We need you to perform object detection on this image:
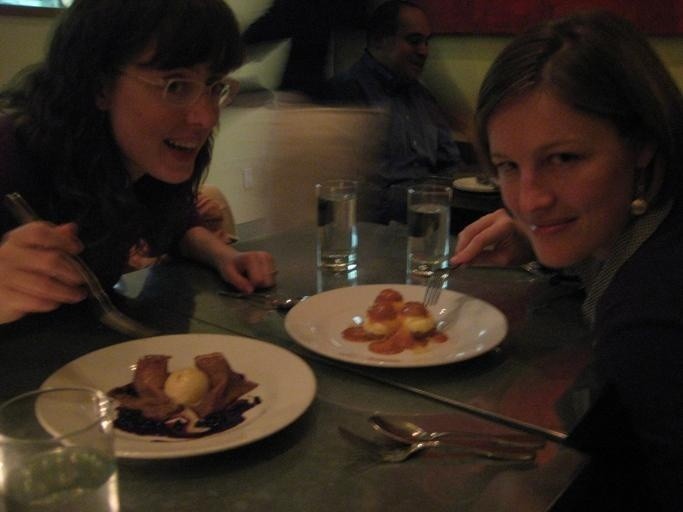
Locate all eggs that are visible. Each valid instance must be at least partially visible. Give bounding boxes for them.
[161,366,212,406]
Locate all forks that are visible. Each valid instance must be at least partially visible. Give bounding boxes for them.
[1,192,158,338]
[423,258,467,305]
[336,424,534,465]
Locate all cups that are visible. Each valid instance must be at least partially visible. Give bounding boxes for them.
[0,386,118,511]
[313,178,360,273]
[404,183,454,279]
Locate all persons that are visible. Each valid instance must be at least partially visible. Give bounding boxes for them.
[319,1,477,231]
[445,9,681,508]
[0,1,277,347]
[240,0,374,93]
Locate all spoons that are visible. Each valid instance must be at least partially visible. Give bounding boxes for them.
[367,414,548,451]
[217,287,299,309]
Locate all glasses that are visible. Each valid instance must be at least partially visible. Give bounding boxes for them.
[122,70,240,109]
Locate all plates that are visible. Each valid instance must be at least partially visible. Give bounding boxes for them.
[35,333,318,459]
[287,282,508,368]
[453,176,498,192]
